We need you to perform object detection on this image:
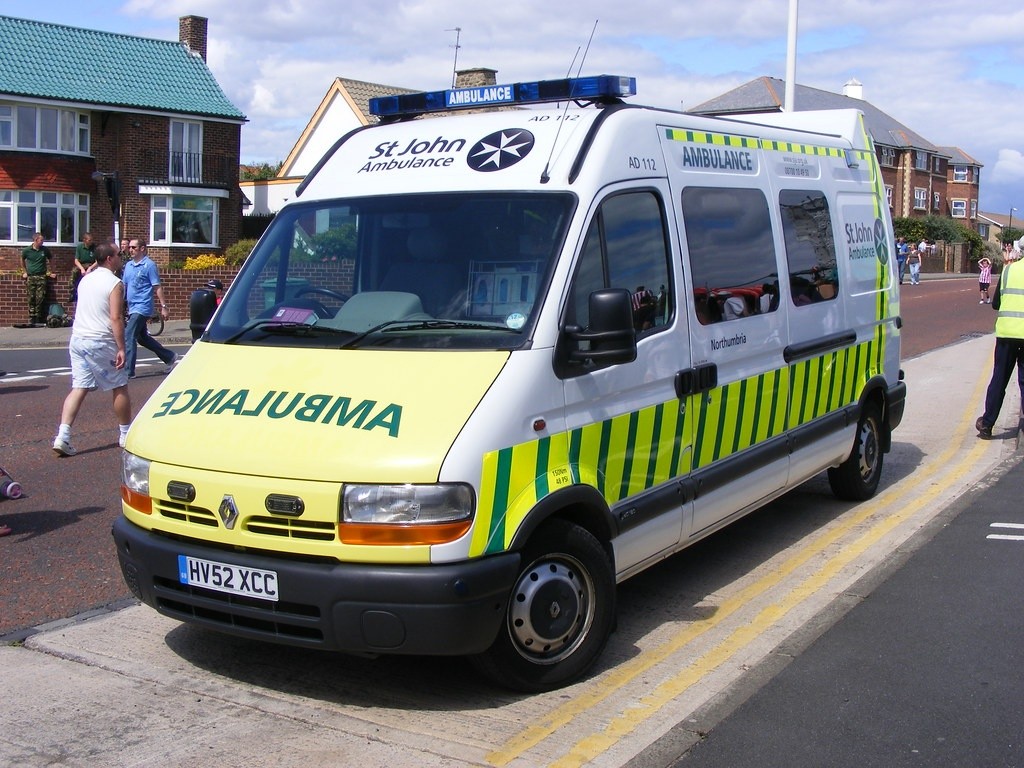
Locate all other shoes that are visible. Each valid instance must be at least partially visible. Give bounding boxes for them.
[916,281,919,285]
[979,300,984,304]
[976,416,993,440]
[910,282,913,285]
[0,524,13,536]
[986,297,990,304]
[161,351,179,373]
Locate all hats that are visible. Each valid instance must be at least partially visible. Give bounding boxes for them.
[523,206,556,226]
[1013,235,1024,257]
[203,279,223,290]
[716,291,728,295]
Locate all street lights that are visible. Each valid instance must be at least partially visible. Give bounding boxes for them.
[1009,207,1018,244]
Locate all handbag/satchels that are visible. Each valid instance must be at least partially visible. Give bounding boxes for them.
[992,275,1002,310]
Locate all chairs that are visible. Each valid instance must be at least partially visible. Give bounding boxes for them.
[377,225,463,311]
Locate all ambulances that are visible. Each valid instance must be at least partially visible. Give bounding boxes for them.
[107,73,908,695]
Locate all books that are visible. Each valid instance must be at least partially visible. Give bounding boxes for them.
[267,307,319,328]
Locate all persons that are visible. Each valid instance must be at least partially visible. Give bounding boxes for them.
[53,241,132,456]
[790,266,837,306]
[631,284,666,333]
[21,232,56,327]
[67,232,133,321]
[976,237,1024,439]
[120,238,178,379]
[203,279,224,307]
[978,242,1023,304]
[918,239,936,258]
[692,280,779,320]
[894,236,921,285]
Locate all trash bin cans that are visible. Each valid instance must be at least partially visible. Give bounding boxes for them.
[262,278,307,310]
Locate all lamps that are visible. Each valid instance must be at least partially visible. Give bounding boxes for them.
[92,169,117,182]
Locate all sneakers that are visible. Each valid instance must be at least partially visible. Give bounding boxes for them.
[118,435,127,448]
[52,437,78,455]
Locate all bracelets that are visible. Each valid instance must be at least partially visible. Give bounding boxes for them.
[161,305,167,308]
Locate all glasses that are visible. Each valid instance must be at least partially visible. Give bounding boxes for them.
[128,245,137,249]
